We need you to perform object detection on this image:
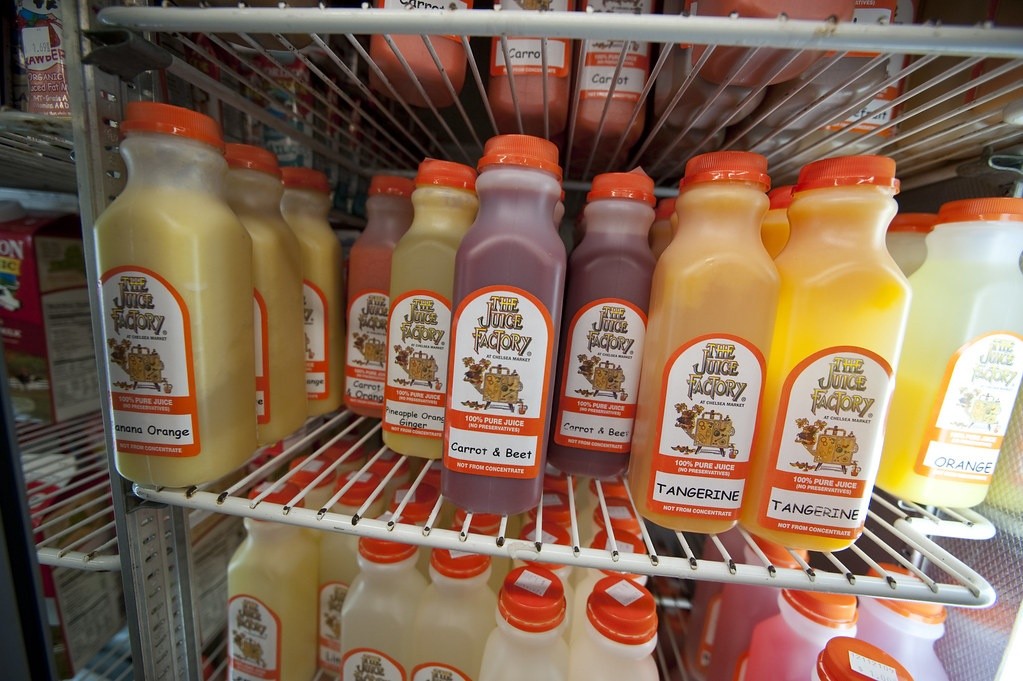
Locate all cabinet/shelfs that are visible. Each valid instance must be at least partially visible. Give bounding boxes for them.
[0,0,1023,681]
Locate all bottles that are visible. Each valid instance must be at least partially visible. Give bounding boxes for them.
[92,0,1023,681]
